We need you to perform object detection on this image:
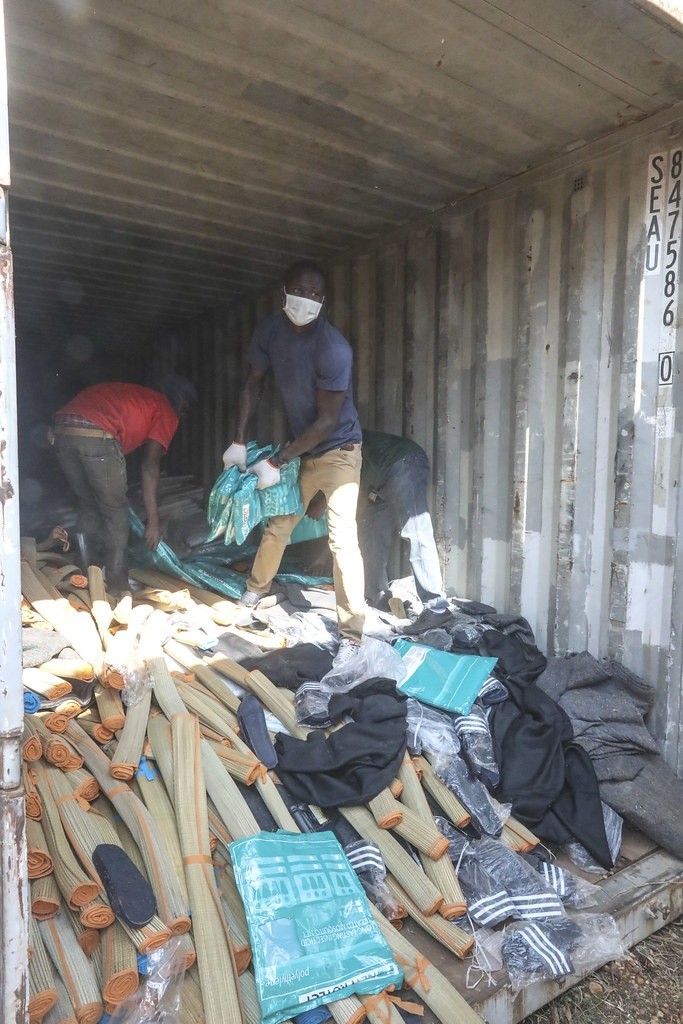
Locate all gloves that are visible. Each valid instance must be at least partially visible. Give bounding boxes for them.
[246,458,282,490]
[222,441,247,473]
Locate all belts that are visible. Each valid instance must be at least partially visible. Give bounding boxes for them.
[53,426,113,438]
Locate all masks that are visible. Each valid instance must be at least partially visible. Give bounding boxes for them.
[281,284,326,327]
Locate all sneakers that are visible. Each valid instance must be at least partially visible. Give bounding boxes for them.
[331,638,359,669]
[241,590,260,605]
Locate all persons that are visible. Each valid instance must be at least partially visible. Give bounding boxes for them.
[305,431,453,637]
[49,373,198,592]
[221,267,362,666]
